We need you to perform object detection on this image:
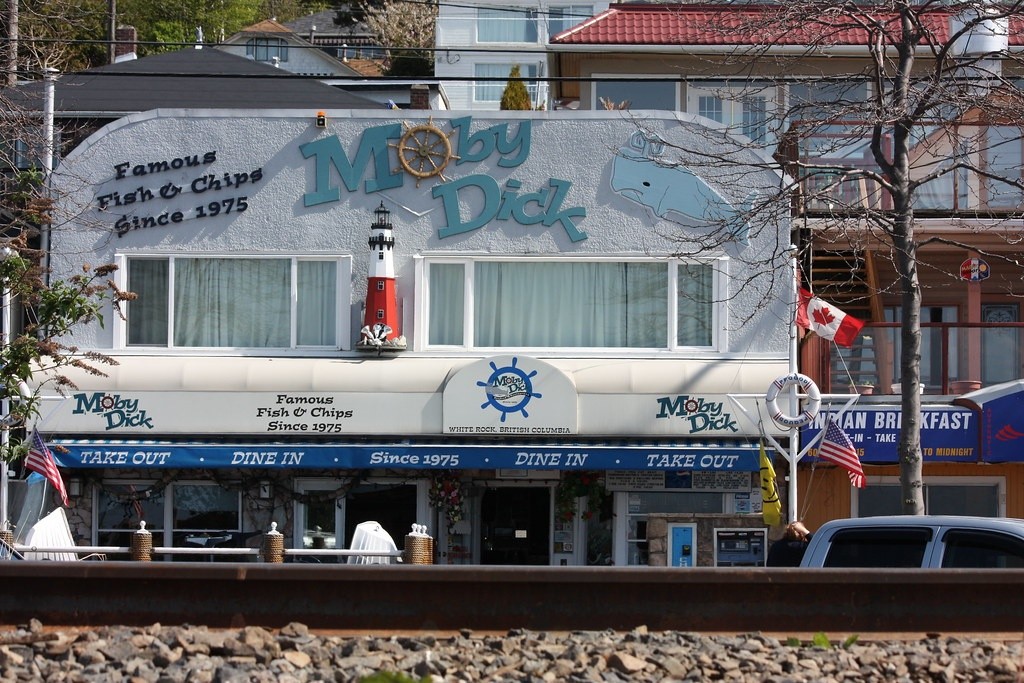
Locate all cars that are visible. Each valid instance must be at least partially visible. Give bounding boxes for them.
[799,514,1024,568]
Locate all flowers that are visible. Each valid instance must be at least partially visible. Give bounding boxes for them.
[554,469,605,523]
[423,468,466,531]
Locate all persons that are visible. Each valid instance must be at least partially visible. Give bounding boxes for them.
[766,522,813,567]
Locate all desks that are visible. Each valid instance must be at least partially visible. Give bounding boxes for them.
[185,534,233,562]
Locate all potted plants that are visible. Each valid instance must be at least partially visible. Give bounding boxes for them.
[848,380,875,395]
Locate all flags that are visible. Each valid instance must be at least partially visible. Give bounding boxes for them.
[760,438,782,527]
[817,418,867,490]
[23,425,70,508]
[128,480,144,519]
[794,287,865,349]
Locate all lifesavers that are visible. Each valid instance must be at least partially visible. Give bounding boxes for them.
[765,373,823,427]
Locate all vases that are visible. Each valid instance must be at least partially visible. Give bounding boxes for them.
[950,380,982,394]
[566,484,589,498]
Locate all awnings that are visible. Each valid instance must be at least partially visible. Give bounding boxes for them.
[45,439,775,474]
